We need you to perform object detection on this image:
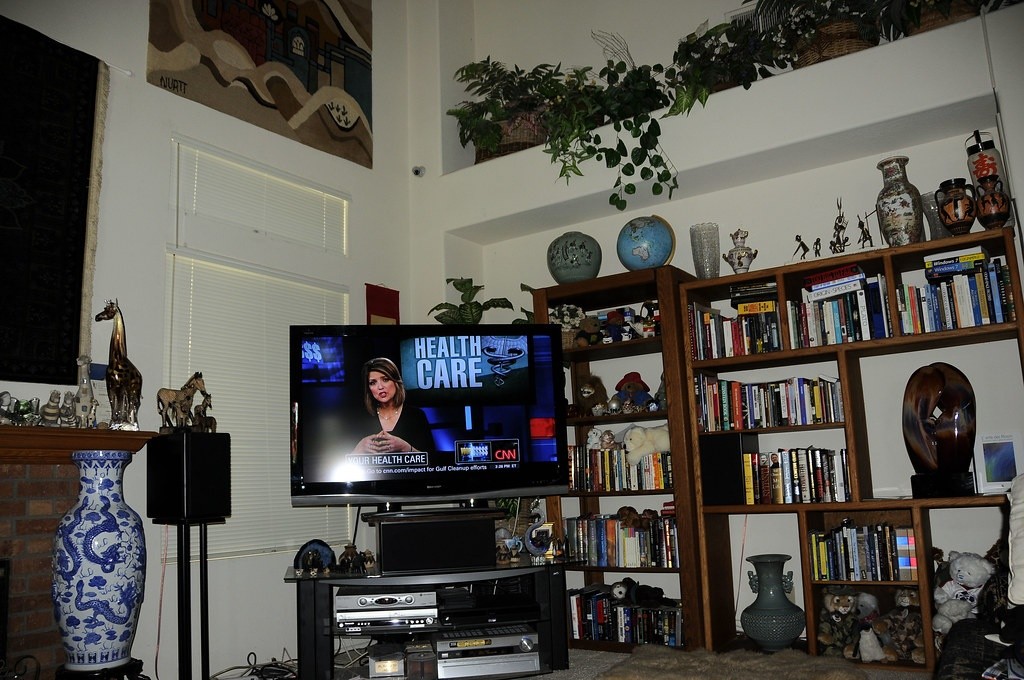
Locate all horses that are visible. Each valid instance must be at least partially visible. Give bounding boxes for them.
[156,372,218,433]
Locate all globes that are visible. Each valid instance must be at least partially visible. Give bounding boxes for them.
[617,215,676,271]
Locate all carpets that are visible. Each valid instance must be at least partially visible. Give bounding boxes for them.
[334,643,933,680]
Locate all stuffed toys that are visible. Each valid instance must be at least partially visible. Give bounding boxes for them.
[617,506,639,529]
[577,376,609,417]
[604,311,632,344]
[587,428,618,449]
[931,549,995,635]
[609,372,655,414]
[572,319,604,347]
[609,577,677,609]
[624,424,671,465]
[640,510,658,530]
[812,585,925,665]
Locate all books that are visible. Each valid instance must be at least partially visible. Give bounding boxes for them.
[687,244,1017,362]
[694,372,844,432]
[567,583,684,647]
[742,444,852,506]
[567,445,673,492]
[807,517,919,583]
[561,499,679,568]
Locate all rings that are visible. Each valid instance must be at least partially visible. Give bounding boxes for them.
[387,445,391,449]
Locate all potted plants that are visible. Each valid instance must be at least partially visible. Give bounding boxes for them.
[446,0,1001,211]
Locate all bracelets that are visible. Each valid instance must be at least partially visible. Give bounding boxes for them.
[411,445,413,452]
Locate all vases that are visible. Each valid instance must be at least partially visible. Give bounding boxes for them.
[874,156,922,248]
[689,222,721,279]
[52,450,147,671]
[740,554,805,651]
[920,190,953,241]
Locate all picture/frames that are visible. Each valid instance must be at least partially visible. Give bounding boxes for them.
[978,432,1021,493]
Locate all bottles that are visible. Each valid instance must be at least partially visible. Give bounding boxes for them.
[875,129,1012,248]
[52,450,147,671]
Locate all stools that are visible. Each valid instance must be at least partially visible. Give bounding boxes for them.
[933,619,1006,680]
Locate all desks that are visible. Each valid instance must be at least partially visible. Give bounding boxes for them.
[0,423,164,680]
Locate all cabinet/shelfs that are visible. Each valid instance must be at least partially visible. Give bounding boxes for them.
[679,226,1024,671]
[531,265,705,654]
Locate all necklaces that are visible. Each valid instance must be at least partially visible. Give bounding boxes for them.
[376,404,398,420]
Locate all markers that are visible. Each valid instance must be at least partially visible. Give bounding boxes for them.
[449,639,492,647]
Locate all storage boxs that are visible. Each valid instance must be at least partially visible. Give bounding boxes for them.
[367,645,437,677]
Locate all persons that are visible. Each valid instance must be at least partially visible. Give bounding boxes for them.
[771,453,779,468]
[343,358,439,469]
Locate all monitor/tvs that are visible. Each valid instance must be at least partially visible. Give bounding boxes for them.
[290,324,569,521]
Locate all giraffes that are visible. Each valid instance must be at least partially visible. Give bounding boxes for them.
[95,298,143,430]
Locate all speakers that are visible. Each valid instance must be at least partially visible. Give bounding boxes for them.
[699,432,759,507]
[146,431,232,521]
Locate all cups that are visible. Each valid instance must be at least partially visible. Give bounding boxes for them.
[918,188,955,240]
[690,223,721,281]
